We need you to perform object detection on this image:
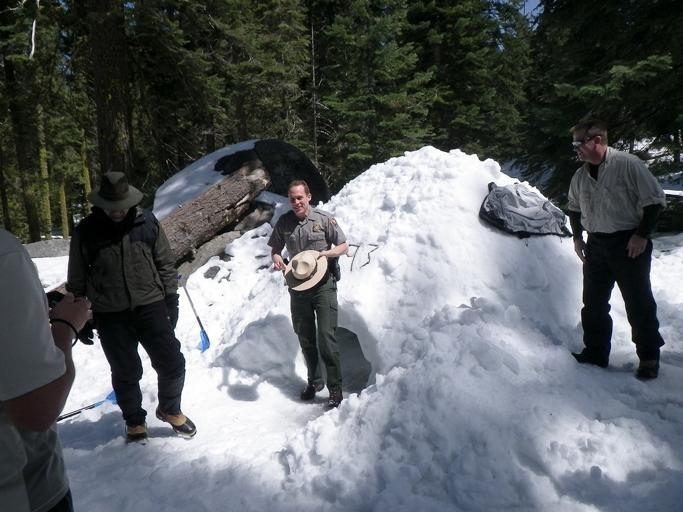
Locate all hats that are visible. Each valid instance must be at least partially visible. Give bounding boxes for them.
[285,250,328,292]
[87,172,143,209]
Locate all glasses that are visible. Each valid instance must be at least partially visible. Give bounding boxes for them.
[573,137,594,148]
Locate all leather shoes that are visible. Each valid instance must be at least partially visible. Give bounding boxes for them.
[328,390,343,406]
[303,381,323,399]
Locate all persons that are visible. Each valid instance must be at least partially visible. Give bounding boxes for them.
[265,180,349,408]
[64,172,196,445]
[566,119,667,382]
[0,227,92,511]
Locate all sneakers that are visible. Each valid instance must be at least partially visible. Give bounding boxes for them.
[637,360,659,379]
[126,425,147,441]
[155,407,197,435]
[572,352,609,367]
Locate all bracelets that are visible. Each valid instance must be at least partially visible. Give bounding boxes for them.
[48,316,79,346]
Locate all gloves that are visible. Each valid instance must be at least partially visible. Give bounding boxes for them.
[164,294,179,328]
[78,322,95,346]
